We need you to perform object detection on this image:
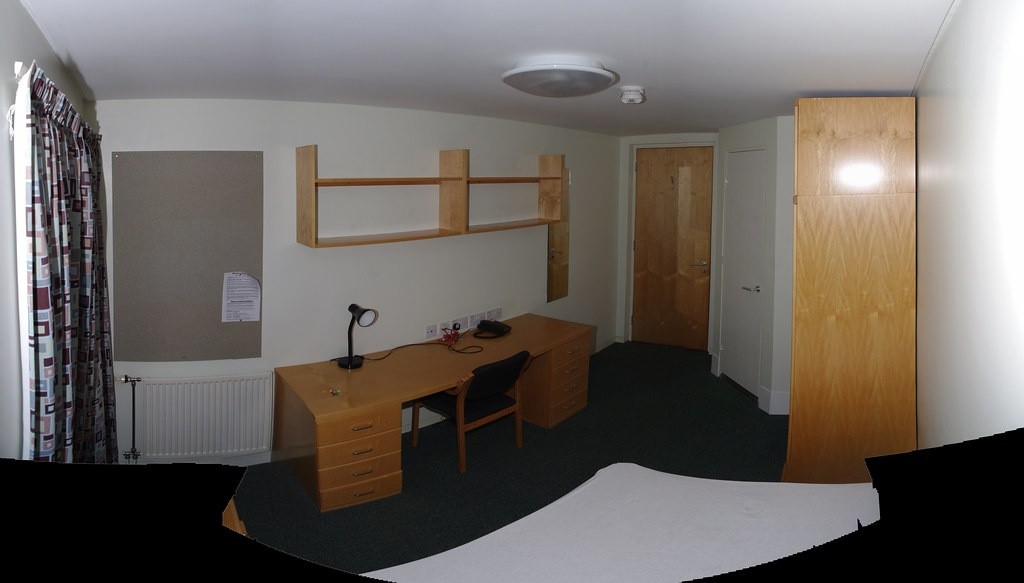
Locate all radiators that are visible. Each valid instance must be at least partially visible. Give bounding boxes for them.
[140,370,275,457]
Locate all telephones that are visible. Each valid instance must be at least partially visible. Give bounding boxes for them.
[477,319,512,335]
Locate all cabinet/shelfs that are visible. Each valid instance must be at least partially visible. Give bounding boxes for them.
[785,96,917,484]
[295,144,565,248]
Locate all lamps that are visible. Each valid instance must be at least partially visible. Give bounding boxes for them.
[501,55,615,98]
[336,303,375,369]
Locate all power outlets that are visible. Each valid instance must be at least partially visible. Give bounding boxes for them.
[451,316,469,330]
[425,323,438,338]
[486,308,502,321]
[469,312,486,327]
[438,321,451,335]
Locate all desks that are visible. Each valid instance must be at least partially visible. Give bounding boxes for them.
[275,313,595,513]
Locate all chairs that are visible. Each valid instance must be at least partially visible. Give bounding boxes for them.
[411,350,534,474]
